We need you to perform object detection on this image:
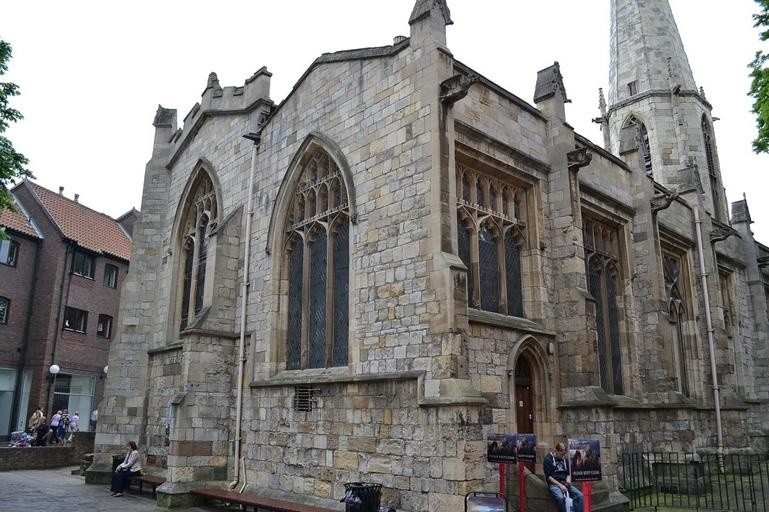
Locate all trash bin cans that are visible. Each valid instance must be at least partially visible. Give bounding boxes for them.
[110,454,132,492]
[339,481,384,512]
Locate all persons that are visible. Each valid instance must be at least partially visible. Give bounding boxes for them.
[27,405,81,446]
[110,440,141,497]
[543,442,584,511]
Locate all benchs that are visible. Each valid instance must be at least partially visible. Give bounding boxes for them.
[129,475,166,499]
[189,489,342,512]
